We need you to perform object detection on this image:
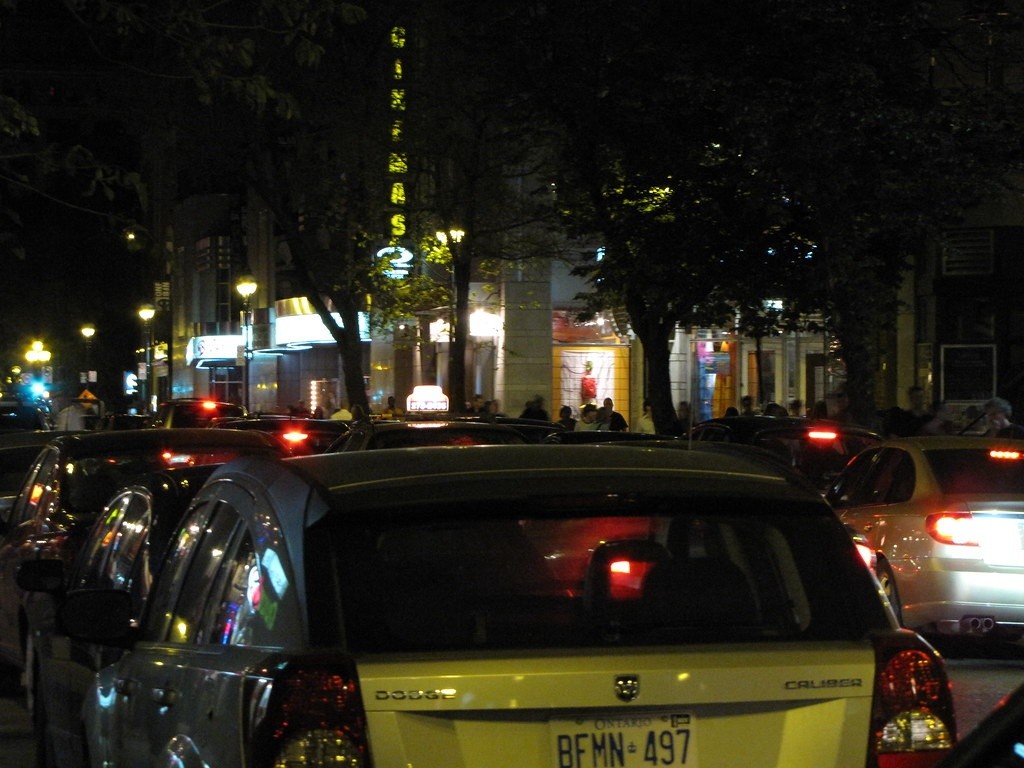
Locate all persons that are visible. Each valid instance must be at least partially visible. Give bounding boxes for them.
[983,397,1024,439]
[518,394,551,422]
[555,404,579,430]
[282,390,337,419]
[882,385,943,438]
[723,406,739,416]
[383,396,406,421]
[603,397,629,432]
[810,399,827,419]
[791,399,802,416]
[579,359,597,402]
[574,403,598,431]
[634,398,655,434]
[587,407,609,430]
[451,395,508,417]
[962,406,987,432]
[762,403,789,417]
[677,400,698,431]
[742,395,758,416]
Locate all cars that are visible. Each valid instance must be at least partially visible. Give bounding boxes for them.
[55,437,962,768]
[0,384,664,768]
[674,411,888,498]
[821,434,1024,647]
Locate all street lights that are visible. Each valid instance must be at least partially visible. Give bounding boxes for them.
[436,226,468,413]
[136,303,160,413]
[79,322,97,390]
[234,276,260,415]
